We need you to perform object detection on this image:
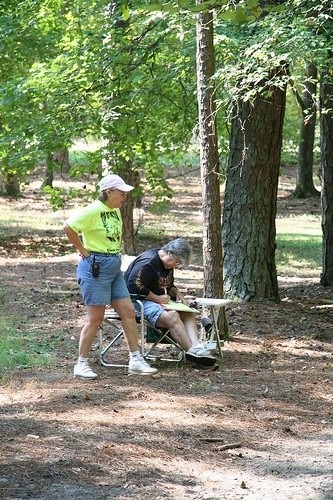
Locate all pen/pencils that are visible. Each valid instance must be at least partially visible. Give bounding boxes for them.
[165,288,167,294]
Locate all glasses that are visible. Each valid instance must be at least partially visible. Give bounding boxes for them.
[175,258,185,269]
[109,192,129,196]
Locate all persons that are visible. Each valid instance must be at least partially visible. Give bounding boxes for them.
[121,238,220,371]
[63,174,158,380]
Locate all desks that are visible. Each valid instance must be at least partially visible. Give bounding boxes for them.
[177,298,230,357]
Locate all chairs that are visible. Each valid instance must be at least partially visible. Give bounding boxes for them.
[98,271,185,368]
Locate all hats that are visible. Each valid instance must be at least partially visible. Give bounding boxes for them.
[100,175,134,192]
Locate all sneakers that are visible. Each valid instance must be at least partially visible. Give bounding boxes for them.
[127,357,158,375]
[73,363,97,379]
[184,346,219,371]
